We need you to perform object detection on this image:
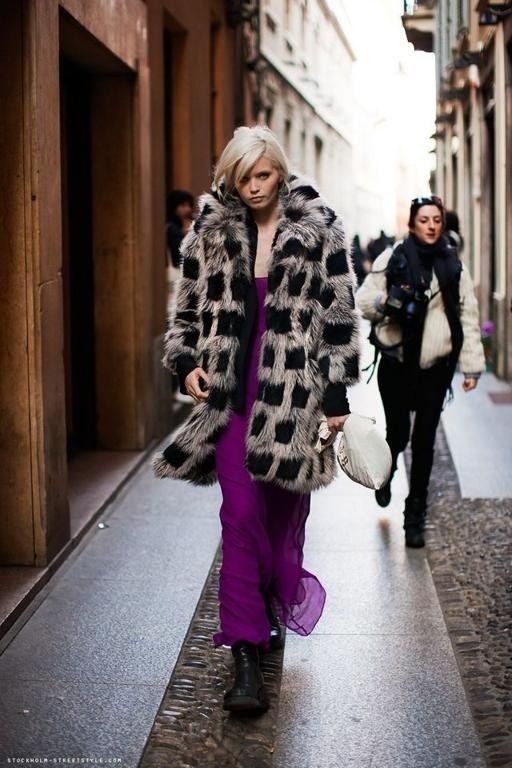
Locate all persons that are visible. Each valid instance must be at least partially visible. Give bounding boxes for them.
[444,210,462,249]
[160,123,362,715]
[349,229,397,289]
[164,185,199,320]
[354,195,489,547]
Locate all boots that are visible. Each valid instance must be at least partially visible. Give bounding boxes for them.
[375,465,398,509]
[222,618,285,713]
[404,498,428,548]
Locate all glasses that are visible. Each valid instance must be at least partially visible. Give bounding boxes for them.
[412,196,443,205]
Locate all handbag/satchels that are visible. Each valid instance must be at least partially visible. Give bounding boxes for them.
[313,414,393,490]
[367,240,407,363]
